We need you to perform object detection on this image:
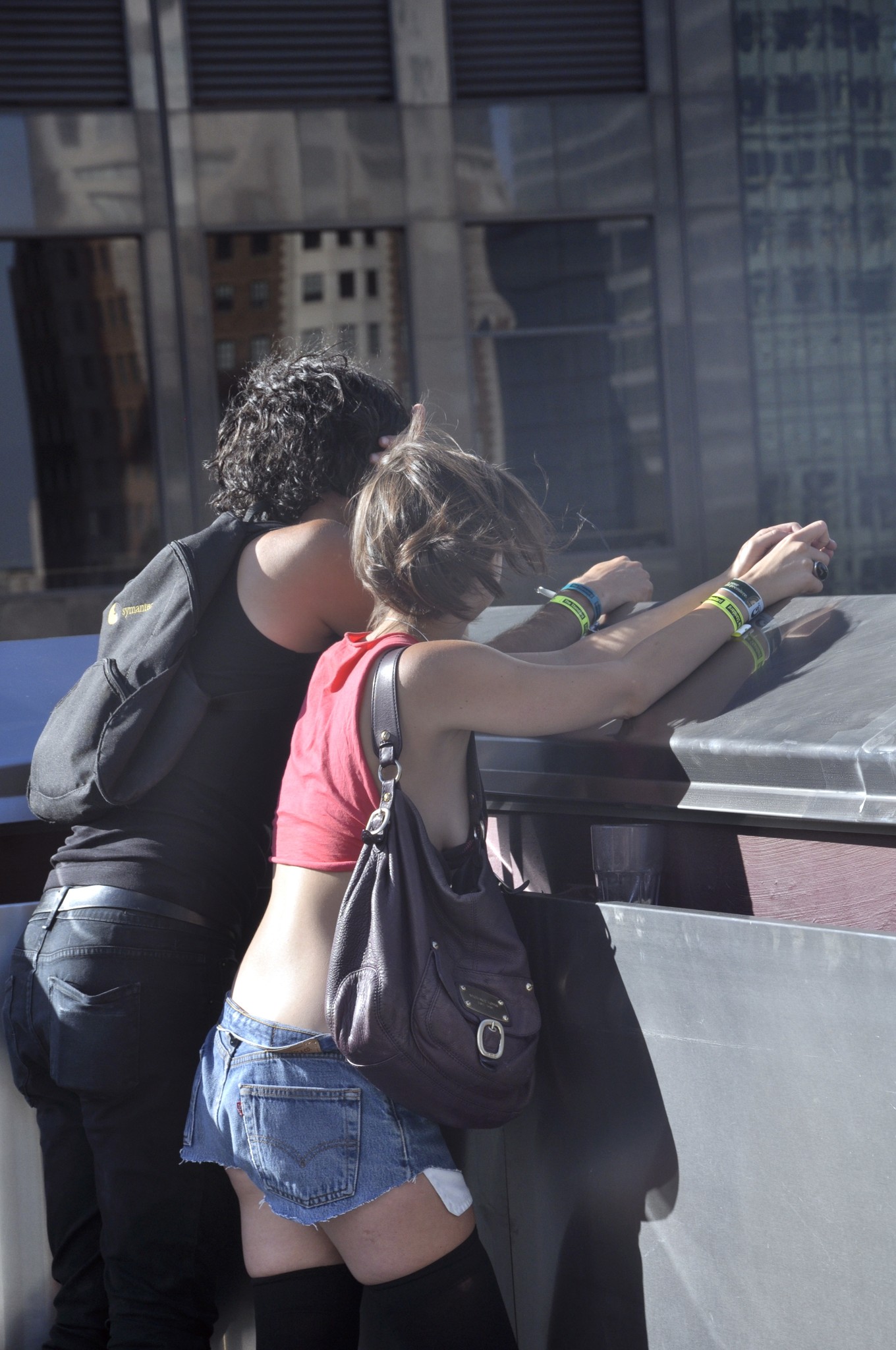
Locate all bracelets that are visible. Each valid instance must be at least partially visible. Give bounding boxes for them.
[702,593,752,637]
[748,612,782,660]
[560,583,601,621]
[548,595,590,638]
[719,577,764,622]
[734,629,765,674]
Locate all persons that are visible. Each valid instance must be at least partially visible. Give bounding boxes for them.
[179,406,838,1350]
[0,341,654,1350]
[623,598,835,743]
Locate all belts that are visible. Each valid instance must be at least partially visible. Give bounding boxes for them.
[28,883,212,925]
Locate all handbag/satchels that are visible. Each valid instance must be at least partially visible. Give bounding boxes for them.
[327,645,540,1130]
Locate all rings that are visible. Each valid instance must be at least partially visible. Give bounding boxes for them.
[812,560,829,584]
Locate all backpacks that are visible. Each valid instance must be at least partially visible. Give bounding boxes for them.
[27,509,274,823]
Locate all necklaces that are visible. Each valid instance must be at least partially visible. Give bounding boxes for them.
[386,613,426,643]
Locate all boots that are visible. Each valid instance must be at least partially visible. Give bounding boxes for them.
[251,1263,358,1350]
[357,1223,519,1350]
